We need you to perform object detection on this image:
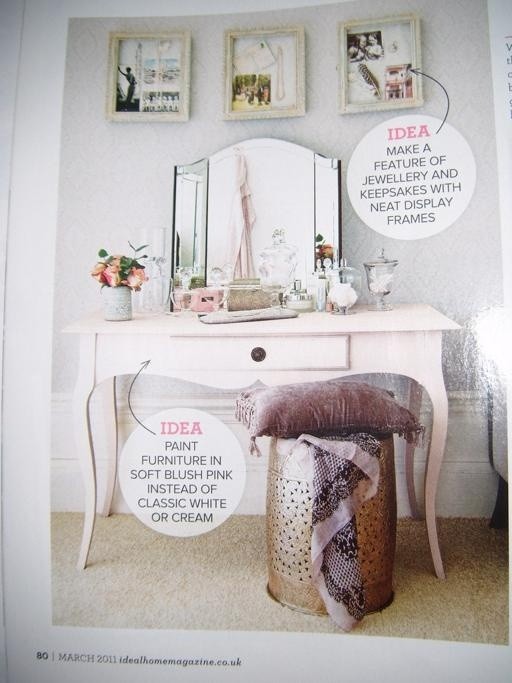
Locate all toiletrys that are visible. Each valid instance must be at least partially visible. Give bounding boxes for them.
[284,256,340,313]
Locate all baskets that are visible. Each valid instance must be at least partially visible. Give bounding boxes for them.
[188,285,277,312]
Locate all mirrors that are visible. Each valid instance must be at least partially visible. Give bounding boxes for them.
[168,137,341,305]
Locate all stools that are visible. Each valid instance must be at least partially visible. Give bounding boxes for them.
[263,435,398,618]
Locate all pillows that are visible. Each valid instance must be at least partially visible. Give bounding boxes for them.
[237,382,425,453]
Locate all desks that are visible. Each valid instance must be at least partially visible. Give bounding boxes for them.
[76,304,462,579]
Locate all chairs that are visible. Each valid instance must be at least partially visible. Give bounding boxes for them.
[469,304,509,530]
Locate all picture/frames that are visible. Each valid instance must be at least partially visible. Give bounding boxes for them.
[338,15,423,114]
[224,29,306,121]
[108,31,192,122]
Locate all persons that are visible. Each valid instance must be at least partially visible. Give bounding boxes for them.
[347,34,368,63]
[363,32,383,61]
[115,63,136,113]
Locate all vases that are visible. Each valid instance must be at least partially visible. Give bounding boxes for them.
[102,286,133,321]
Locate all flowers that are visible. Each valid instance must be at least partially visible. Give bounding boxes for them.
[90,240,149,292]
